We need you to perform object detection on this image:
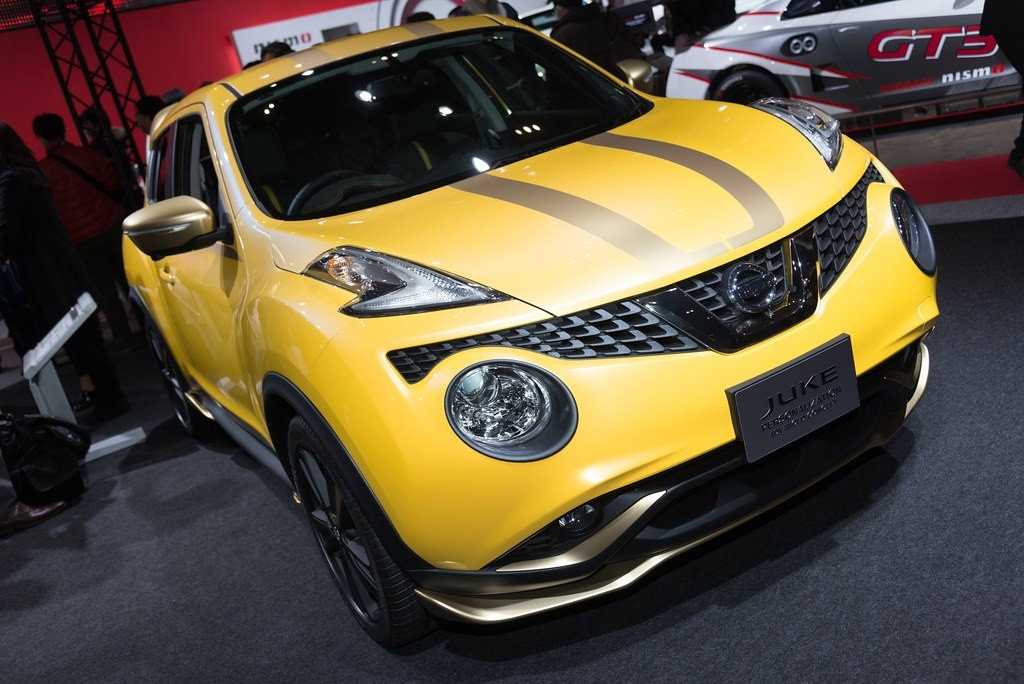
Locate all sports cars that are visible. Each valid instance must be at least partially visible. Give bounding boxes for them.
[666,1,1021,143]
[120,12,944,659]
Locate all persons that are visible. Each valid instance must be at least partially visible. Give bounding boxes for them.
[1,42,293,427]
[979,0,1024,174]
[408,0,737,84]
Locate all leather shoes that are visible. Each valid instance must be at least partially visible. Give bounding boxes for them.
[0,501,67,538]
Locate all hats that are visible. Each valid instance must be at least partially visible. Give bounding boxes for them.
[547,0,583,7]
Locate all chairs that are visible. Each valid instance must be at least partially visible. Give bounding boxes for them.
[255,108,383,215]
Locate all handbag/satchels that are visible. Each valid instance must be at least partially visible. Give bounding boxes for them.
[0,413,92,505]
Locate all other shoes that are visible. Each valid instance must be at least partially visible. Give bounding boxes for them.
[73,389,97,412]
